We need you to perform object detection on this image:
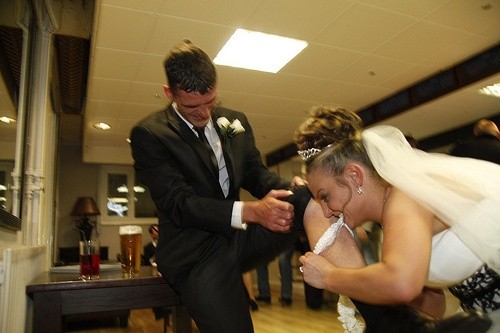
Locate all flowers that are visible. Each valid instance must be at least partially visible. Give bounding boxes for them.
[217,117,245,152]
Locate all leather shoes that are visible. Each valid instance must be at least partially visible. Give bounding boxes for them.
[364,313,493,333]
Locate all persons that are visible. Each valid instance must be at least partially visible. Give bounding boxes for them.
[129,40,500,333]
[293,105,500,333]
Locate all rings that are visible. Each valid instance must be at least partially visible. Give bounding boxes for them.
[299,265,305,274]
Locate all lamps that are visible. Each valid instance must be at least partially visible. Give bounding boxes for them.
[70,196,101,242]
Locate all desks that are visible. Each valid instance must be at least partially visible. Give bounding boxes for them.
[26,263,193,333]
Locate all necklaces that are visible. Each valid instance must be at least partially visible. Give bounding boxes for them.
[379,183,390,228]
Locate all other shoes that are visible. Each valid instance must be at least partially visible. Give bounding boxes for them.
[256,297,270,301]
[282,296,291,304]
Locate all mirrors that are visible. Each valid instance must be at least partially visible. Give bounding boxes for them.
[0,0,39,232]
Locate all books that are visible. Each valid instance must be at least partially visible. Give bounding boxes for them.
[51,265,122,273]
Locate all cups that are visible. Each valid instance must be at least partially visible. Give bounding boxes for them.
[79,240,100,279]
[119,225,143,278]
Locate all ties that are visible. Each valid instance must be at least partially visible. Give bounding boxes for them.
[192,126,219,185]
